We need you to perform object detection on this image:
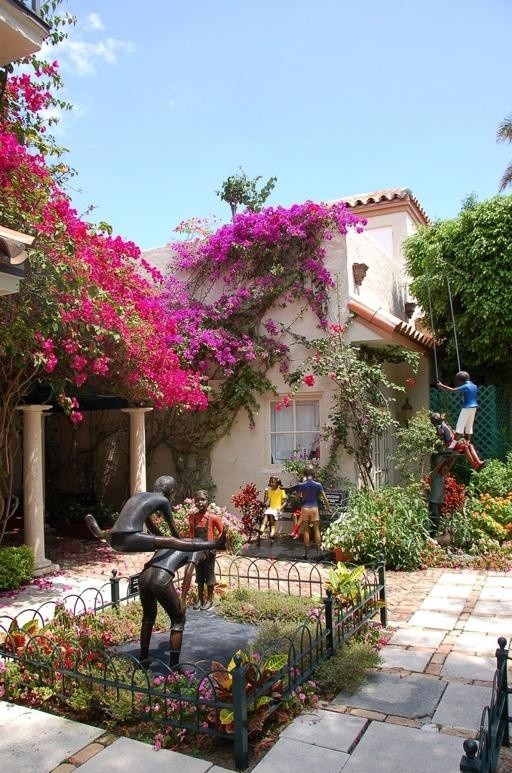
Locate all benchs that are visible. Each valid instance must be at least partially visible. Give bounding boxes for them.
[257,485,348,550]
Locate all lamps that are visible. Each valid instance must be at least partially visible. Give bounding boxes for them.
[401,396,413,429]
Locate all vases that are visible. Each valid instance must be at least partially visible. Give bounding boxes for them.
[334,547,353,566]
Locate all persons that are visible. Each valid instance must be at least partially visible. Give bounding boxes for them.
[139,537,213,674]
[84,475,227,553]
[188,490,223,611]
[431,412,487,472]
[288,476,307,540]
[279,463,332,562]
[436,370,478,442]
[427,456,457,538]
[258,477,288,539]
